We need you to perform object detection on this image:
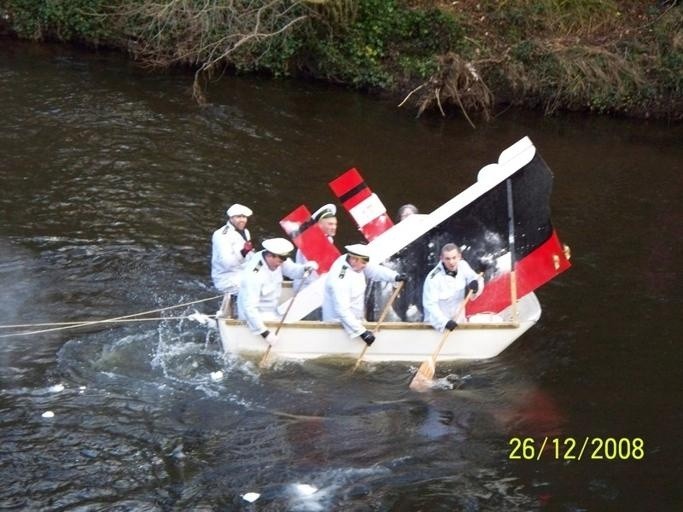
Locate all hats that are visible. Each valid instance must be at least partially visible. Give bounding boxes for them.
[262,238,294,256]
[344,244,373,258]
[311,203,336,222]
[227,203,253,217]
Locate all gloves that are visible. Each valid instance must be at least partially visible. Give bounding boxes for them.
[360,331,375,345]
[395,273,408,282]
[445,320,457,329]
[305,262,318,271]
[266,332,276,345]
[470,281,478,293]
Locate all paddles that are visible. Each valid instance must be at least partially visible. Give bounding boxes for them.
[258,267,313,369]
[407,264,487,392]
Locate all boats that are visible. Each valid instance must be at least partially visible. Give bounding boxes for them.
[215,277,541,361]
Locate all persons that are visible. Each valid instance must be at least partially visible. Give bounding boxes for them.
[322,243,408,346]
[209,203,256,296]
[236,237,320,346]
[422,243,485,333]
[293,203,338,296]
[394,203,418,223]
